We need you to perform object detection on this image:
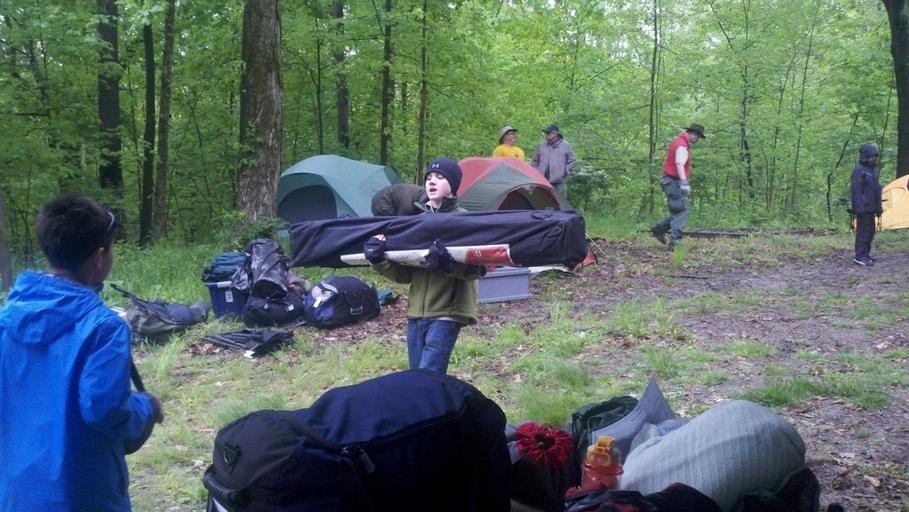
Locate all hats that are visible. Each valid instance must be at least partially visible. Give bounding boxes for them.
[498,125,518,144]
[541,124,559,133]
[424,157,462,195]
[683,124,705,138]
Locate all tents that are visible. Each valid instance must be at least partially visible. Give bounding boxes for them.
[853,173,909,231]
[273,151,407,226]
[449,156,599,275]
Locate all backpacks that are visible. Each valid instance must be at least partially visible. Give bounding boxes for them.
[304,273,380,329]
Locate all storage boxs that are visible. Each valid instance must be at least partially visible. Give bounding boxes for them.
[205,280,249,322]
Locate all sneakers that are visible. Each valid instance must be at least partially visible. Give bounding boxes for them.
[651,227,666,244]
[853,256,875,266]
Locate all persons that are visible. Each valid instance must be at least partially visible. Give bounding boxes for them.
[1,193,165,512]
[492,125,526,164]
[530,124,578,201]
[362,156,489,377]
[648,122,708,252]
[846,143,883,268]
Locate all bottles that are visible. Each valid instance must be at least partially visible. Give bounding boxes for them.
[579,434,625,491]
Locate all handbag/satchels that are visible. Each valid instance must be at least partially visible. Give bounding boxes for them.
[202,236,308,327]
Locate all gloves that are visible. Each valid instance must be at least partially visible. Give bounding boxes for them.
[679,180,691,196]
